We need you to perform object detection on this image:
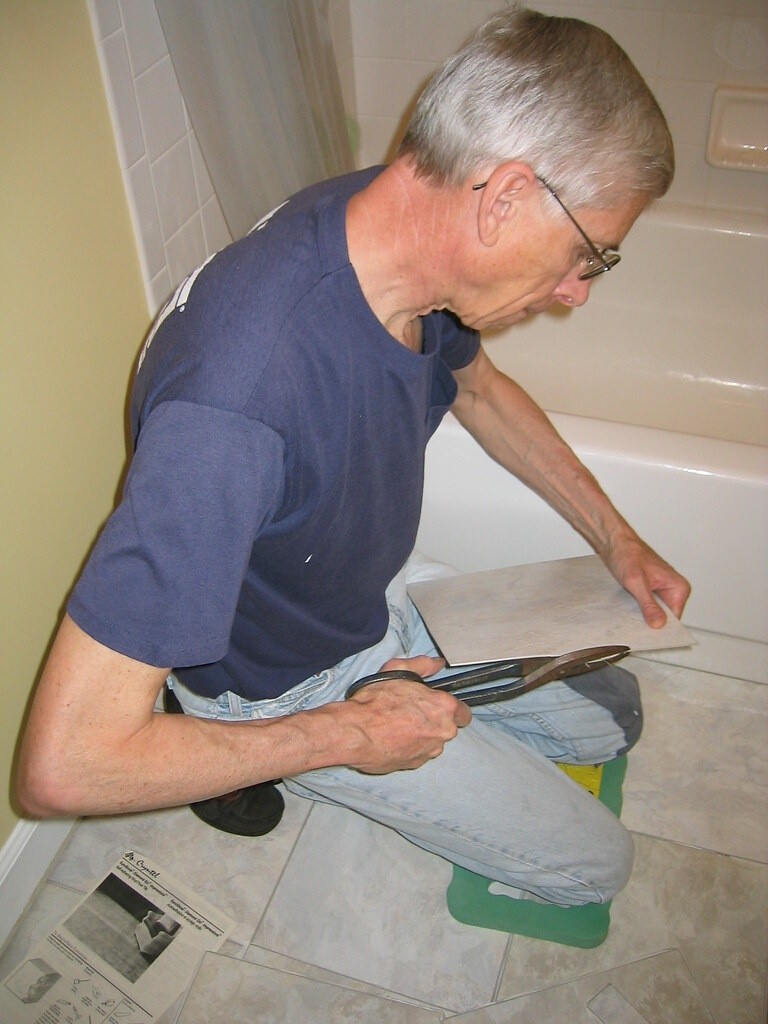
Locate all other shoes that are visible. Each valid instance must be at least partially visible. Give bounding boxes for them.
[165,686,284,836]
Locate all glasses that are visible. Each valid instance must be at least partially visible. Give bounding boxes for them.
[472,176,621,280]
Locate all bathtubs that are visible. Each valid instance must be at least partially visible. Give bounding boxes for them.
[412,404,768,688]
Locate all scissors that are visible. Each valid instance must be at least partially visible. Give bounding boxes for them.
[341,644,632,709]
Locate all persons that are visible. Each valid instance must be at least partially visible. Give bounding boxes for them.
[16,6,692,908]
